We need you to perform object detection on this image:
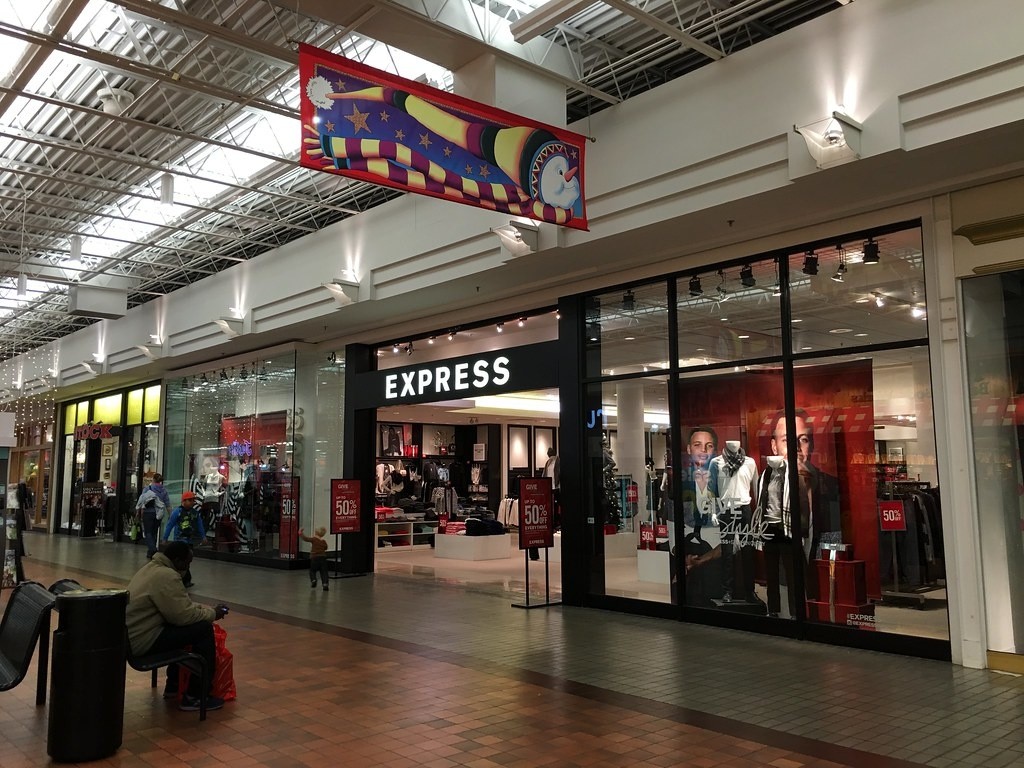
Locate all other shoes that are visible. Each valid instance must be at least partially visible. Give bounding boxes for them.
[790,616,796,620]
[184,582,194,588]
[322,583,329,591]
[147,548,158,560]
[769,612,779,618]
[311,580,317,588]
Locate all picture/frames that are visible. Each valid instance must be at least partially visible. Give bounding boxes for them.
[380,425,405,457]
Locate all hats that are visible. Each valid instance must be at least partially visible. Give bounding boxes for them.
[181,492,197,501]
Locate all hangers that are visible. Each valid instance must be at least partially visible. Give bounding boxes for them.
[879,482,913,500]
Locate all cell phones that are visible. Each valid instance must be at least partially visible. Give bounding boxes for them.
[221,606,229,614]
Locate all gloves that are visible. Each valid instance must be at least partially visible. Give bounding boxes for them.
[214,604,230,619]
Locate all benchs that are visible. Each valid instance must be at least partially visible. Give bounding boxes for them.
[50,579,210,723]
[0,580,60,705]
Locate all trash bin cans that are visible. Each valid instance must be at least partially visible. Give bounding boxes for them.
[80,506,100,537]
[46,586,132,765]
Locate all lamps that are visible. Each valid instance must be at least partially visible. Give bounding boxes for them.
[181,357,269,391]
[322,278,361,305]
[551,309,562,321]
[211,316,244,338]
[447,331,456,340]
[41,375,57,389]
[907,302,922,318]
[82,359,102,376]
[801,250,821,277]
[792,111,863,169]
[714,269,730,304]
[427,336,436,345]
[137,343,163,360]
[860,237,884,266]
[587,305,602,343]
[157,163,177,211]
[621,288,637,311]
[68,213,84,265]
[687,275,703,298]
[518,315,529,327]
[488,220,538,257]
[390,343,403,354]
[496,322,505,332]
[772,257,781,297]
[16,197,27,297]
[405,342,414,356]
[872,293,886,309]
[739,262,760,288]
[829,246,851,285]
[5,387,20,397]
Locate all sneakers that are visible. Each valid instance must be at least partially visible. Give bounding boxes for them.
[178,691,225,711]
[163,687,178,703]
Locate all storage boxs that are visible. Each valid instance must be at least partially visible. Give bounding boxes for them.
[820,543,853,560]
[807,601,876,632]
[817,558,870,603]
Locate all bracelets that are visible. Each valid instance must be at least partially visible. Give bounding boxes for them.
[299,531,302,535]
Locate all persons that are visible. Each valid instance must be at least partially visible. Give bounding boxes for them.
[136,472,172,559]
[190,451,219,511]
[381,470,404,506]
[756,455,806,619]
[202,467,226,527]
[84,494,101,508]
[602,449,616,488]
[542,456,560,526]
[770,408,841,542]
[127,540,226,709]
[653,469,667,524]
[681,427,718,528]
[162,491,207,588]
[707,440,759,604]
[424,458,449,481]
[298,527,330,591]
[387,427,400,452]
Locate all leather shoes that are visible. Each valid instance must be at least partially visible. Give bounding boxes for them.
[744,590,764,604]
[722,592,734,603]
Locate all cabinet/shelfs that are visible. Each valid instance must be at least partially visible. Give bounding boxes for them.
[375,520,440,555]
